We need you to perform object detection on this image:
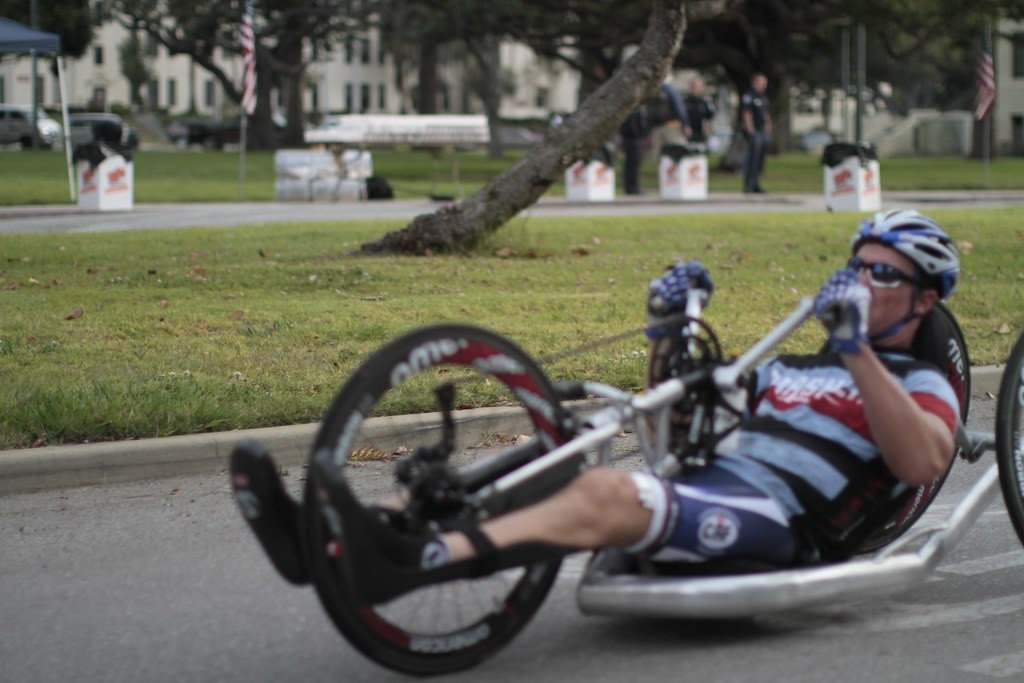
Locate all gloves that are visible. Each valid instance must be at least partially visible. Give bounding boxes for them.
[814,270,871,353]
[646,260,714,338]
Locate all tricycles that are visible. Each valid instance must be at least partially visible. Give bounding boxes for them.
[292,266,1023,683]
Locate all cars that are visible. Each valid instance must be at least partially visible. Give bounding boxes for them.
[167,116,243,152]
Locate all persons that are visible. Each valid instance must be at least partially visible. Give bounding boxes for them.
[571,73,774,195]
[226,210,960,591]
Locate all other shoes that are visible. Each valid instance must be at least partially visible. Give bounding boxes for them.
[309,453,446,604]
[229,442,314,586]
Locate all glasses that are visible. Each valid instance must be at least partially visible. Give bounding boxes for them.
[845,256,923,288]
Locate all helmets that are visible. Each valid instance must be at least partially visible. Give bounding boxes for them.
[849,207,960,299]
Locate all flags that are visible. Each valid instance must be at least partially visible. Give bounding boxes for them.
[239,3,259,115]
[967,25,999,123]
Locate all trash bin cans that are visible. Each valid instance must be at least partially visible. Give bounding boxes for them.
[563,144,616,203]
[73,141,134,212]
[822,143,882,214]
[658,144,709,201]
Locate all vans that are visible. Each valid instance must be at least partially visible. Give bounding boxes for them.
[0,103,62,151]
